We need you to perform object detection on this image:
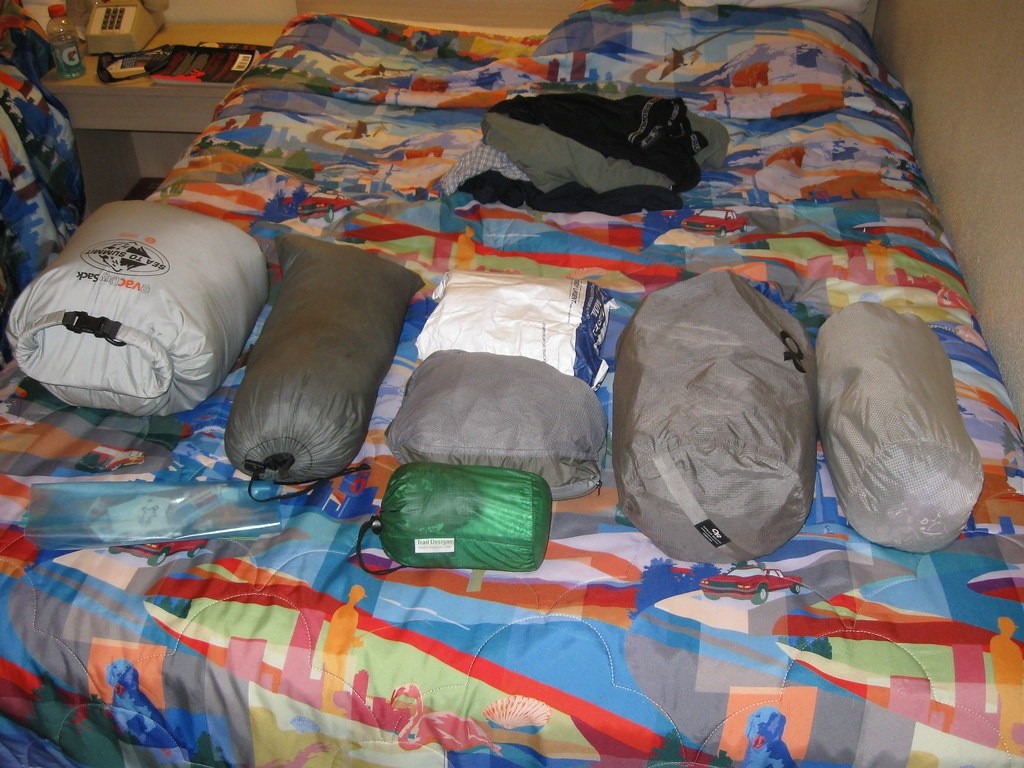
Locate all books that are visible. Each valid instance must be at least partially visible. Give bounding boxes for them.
[150,41,273,86]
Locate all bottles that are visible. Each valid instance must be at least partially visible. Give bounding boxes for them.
[47,5,84,80]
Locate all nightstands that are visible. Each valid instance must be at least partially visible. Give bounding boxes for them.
[40,19,287,221]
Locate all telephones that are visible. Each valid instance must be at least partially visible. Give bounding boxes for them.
[86,0,169,55]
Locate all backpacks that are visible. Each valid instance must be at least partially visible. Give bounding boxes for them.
[0,0,87,315]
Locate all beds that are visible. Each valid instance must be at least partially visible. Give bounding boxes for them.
[0,0,1024,768]
